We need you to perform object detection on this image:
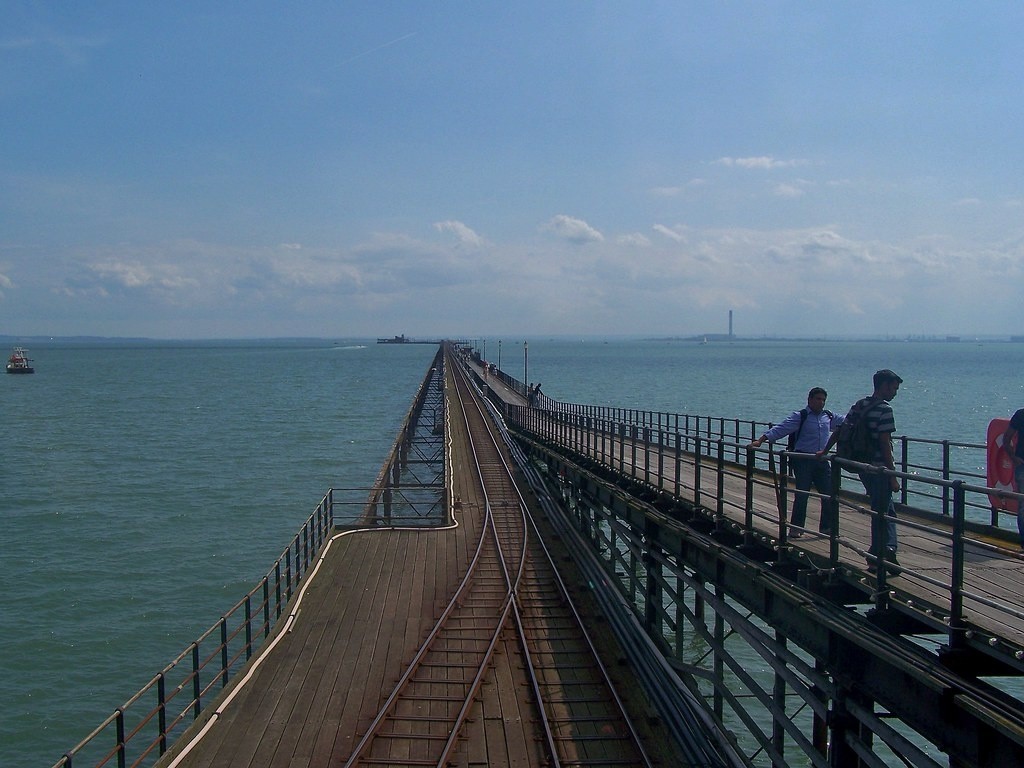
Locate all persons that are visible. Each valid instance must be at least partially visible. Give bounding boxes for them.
[816,369,903,575]
[1003,410,1024,553]
[457,348,471,363]
[527,383,545,409]
[483,361,497,382]
[750,387,846,537]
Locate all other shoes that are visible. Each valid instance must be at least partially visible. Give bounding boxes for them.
[789,527,801,538]
[819,532,843,539]
[868,546,901,567]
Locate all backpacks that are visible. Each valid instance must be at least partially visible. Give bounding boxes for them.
[787,409,833,476]
[837,399,891,462]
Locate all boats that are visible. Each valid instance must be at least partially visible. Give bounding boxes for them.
[377,335,410,344]
[6,346,35,373]
